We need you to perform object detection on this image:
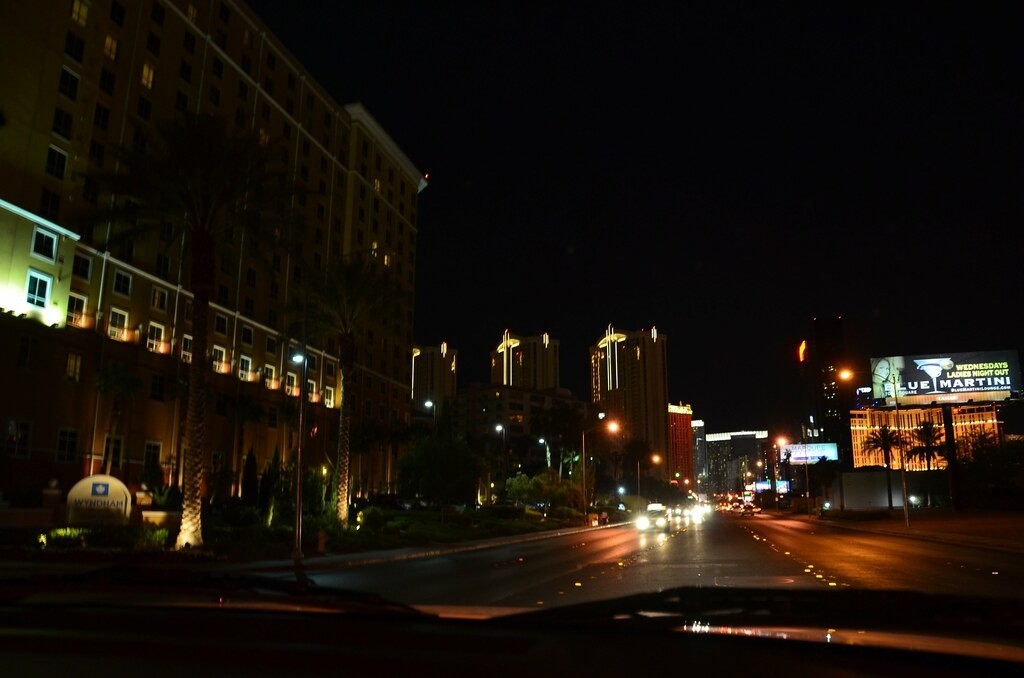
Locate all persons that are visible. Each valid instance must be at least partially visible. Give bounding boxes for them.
[871,356,905,397]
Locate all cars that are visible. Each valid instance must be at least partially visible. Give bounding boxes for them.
[637,510,670,534]
[730,505,740,513]
[741,504,754,516]
[753,505,762,514]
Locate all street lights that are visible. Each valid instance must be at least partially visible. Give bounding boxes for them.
[637,455,660,497]
[495,424,505,449]
[582,420,619,526]
[778,437,810,518]
[292,351,307,562]
[425,400,436,433]
[838,368,910,528]
